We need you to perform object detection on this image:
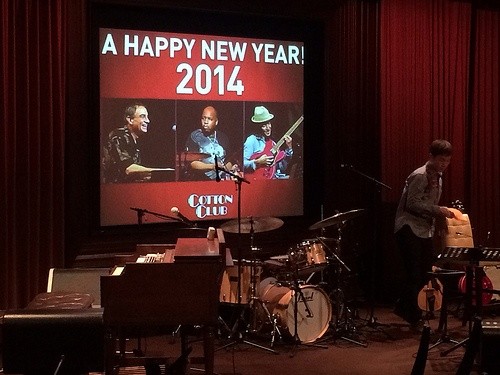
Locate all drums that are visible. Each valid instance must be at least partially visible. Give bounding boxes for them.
[270,255,292,281]
[249,277,332,344]
[288,244,330,276]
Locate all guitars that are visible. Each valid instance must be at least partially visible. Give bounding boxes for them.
[245,116,304,180]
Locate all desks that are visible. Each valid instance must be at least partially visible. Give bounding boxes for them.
[27,292,94,312]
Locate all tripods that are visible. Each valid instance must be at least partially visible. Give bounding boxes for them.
[414,276,467,353]
[276,260,328,356]
[312,224,368,348]
[216,168,279,353]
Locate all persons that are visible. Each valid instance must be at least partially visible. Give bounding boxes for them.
[244,105,294,179]
[183,106,243,181]
[394,139,457,332]
[102,103,153,183]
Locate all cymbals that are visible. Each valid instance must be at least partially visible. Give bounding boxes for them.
[220,215,284,234]
[309,210,364,230]
[305,237,338,242]
[177,152,211,162]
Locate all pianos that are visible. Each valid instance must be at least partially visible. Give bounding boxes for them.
[100,228,234,375]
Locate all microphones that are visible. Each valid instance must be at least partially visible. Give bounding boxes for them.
[215,155,221,182]
[173,125,183,140]
[171,207,194,227]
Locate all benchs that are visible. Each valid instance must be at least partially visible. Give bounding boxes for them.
[0,310,105,375]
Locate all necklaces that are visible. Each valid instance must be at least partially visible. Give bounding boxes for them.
[204,128,216,145]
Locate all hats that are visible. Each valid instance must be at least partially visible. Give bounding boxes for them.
[251,106,274,123]
